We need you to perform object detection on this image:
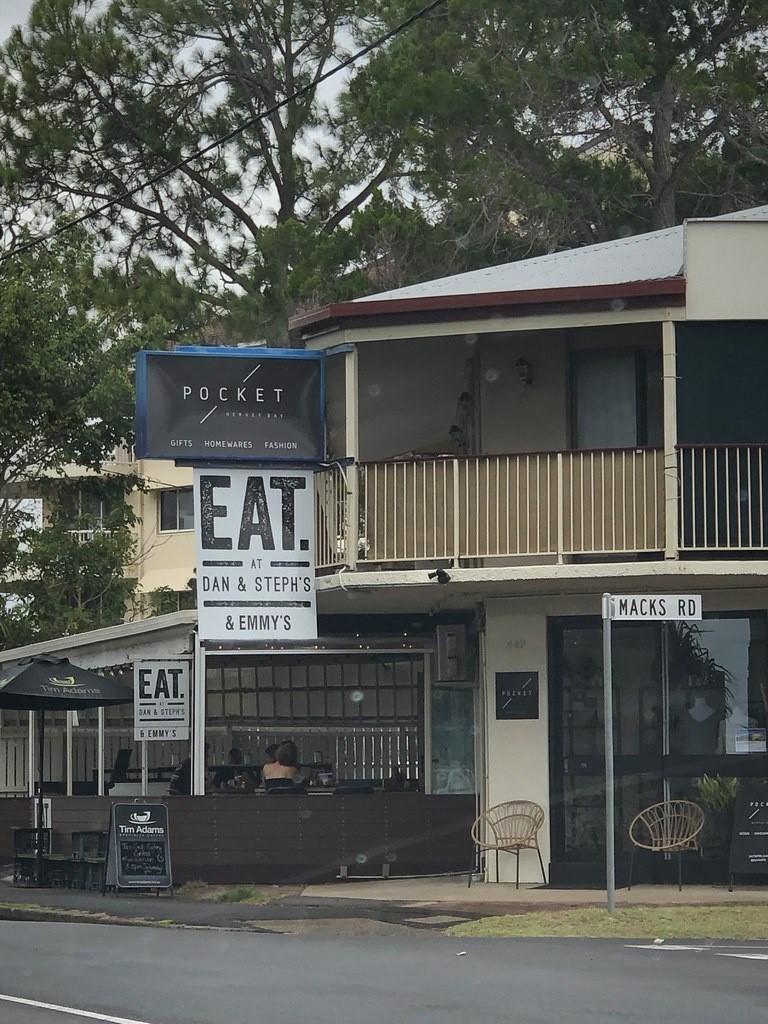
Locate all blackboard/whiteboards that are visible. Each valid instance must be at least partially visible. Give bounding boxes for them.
[118,841,167,875]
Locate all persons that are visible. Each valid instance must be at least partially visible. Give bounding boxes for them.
[716,704,761,757]
[214,749,259,791]
[265,744,278,764]
[673,693,721,758]
[169,744,219,796]
[259,740,300,793]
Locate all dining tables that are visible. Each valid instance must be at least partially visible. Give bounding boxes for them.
[209,786,382,792]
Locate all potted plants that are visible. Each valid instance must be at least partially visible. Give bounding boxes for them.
[695,773,740,849]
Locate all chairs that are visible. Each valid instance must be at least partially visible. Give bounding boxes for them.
[628,799,706,891]
[48,829,80,888]
[467,799,548,890]
[10,826,50,887]
[68,801,116,892]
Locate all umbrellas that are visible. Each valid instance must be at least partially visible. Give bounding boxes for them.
[0,655,135,881]
